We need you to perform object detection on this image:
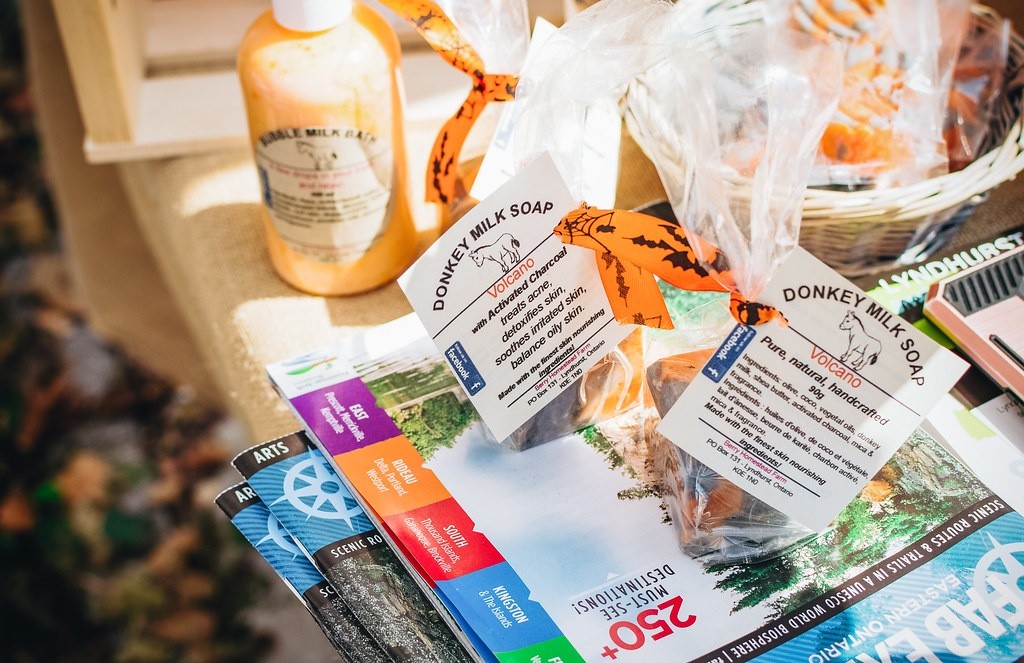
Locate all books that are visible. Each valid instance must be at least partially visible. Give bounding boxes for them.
[214,276,1023,662]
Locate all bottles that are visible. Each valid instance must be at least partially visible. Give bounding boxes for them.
[237,1,417,298]
